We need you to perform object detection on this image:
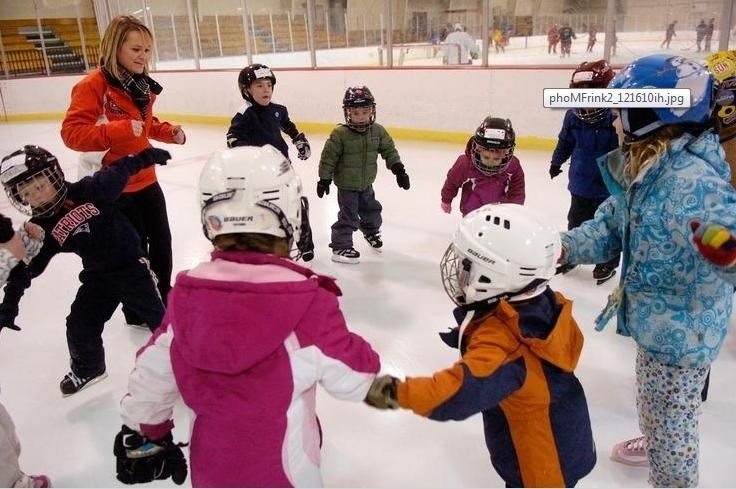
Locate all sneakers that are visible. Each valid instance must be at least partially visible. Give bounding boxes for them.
[60,366,105,394]
[303,250,314,261]
[333,249,360,257]
[17,475,50,488]
[613,437,646,466]
[593,254,619,280]
[364,232,383,247]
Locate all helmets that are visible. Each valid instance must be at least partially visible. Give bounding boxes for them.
[568,61,615,124]
[470,115,515,176]
[200,145,303,243]
[703,49,735,126]
[1,145,68,220]
[239,63,277,96]
[439,203,560,307]
[344,86,378,131]
[607,53,712,137]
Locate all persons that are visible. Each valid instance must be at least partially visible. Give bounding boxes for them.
[1,15,735,488]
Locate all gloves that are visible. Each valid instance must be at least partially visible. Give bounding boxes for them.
[317,180,332,198]
[113,419,187,485]
[442,201,451,213]
[550,165,563,179]
[364,374,399,410]
[0,289,24,332]
[137,148,171,169]
[691,222,736,266]
[392,163,410,190]
[292,131,311,161]
